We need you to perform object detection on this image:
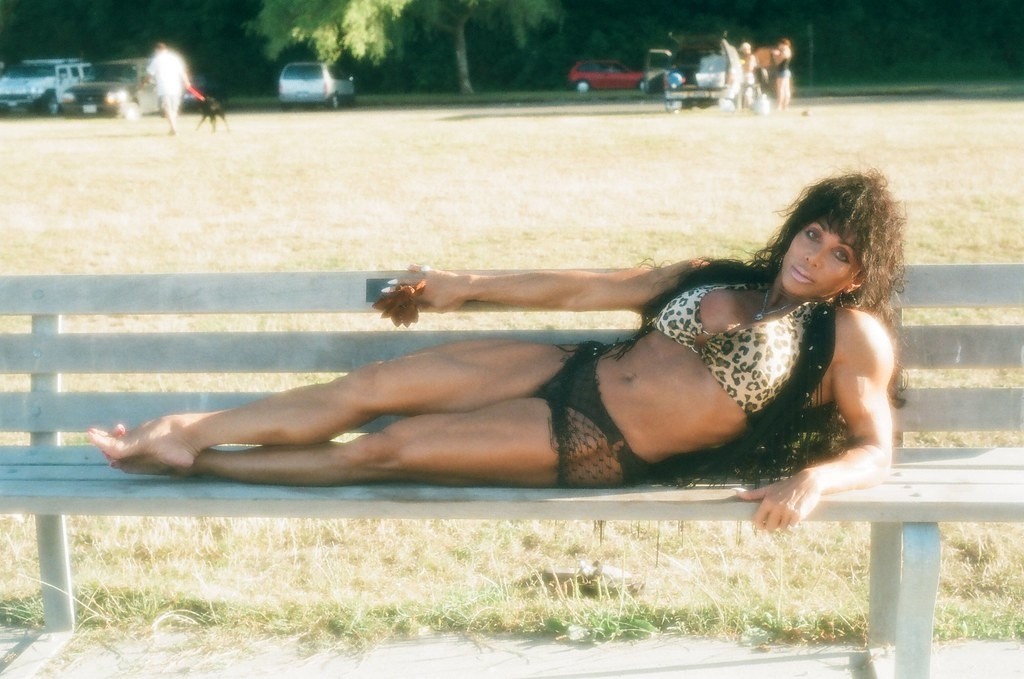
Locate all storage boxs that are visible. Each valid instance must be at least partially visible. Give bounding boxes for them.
[694,71,725,89]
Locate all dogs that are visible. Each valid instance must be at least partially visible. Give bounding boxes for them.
[195,96,228,134]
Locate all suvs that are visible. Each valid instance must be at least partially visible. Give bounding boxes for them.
[660,41,734,109]
[567,59,650,94]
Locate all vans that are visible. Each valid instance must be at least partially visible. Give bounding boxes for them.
[0,60,97,116]
[277,62,357,109]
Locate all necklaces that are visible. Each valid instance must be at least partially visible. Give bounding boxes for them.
[755,289,791,321]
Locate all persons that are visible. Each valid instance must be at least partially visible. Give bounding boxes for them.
[741,37,793,108]
[106,42,190,135]
[87,172,905,529]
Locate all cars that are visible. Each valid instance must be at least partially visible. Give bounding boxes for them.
[62,60,161,119]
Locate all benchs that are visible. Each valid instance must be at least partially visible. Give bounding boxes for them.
[0,264,1024,679]
